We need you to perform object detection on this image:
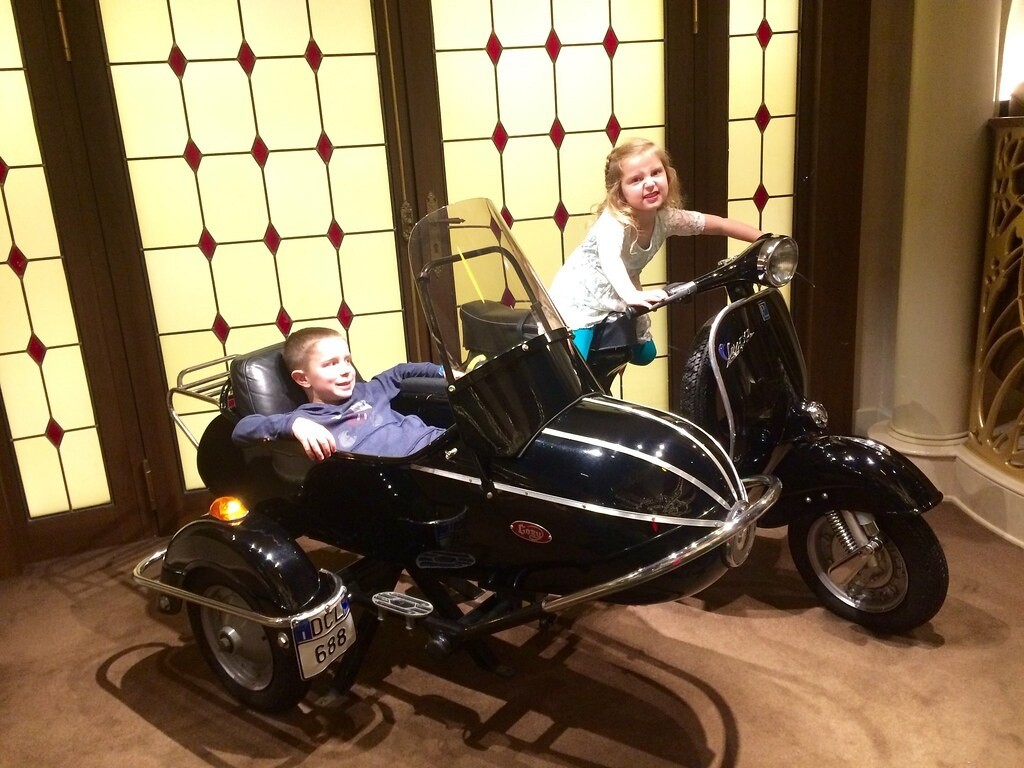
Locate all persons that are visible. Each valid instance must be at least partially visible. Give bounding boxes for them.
[536,138,767,365]
[231,325,466,457]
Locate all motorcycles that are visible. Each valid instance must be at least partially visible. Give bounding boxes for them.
[132,197,950,716]
